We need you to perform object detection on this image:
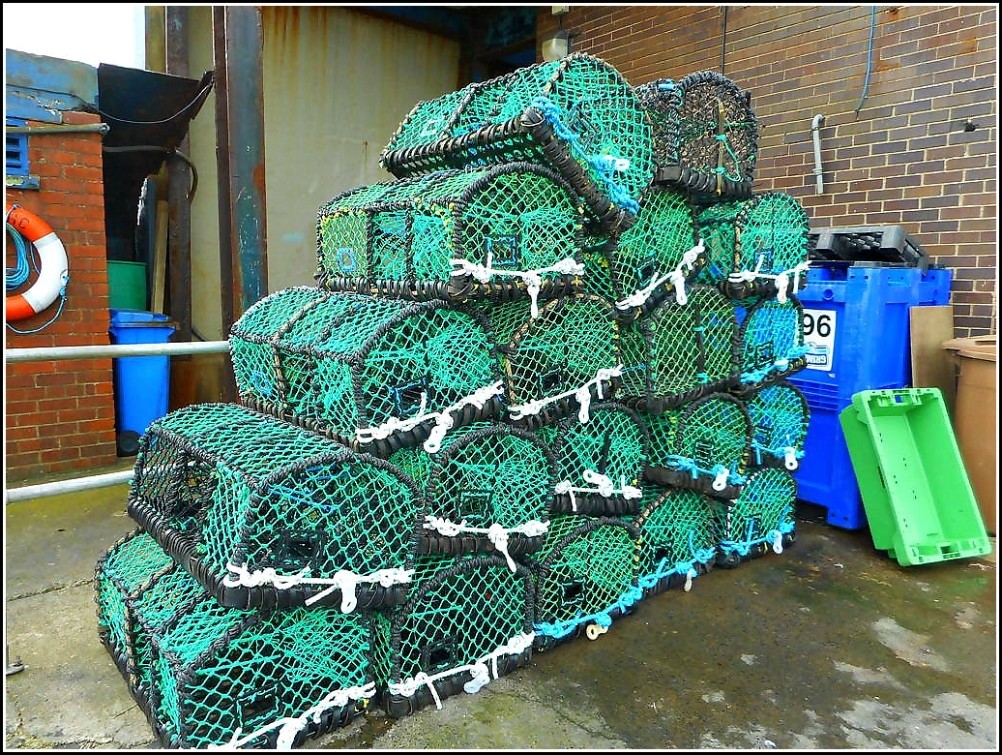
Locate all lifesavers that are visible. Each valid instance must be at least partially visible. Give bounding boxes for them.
[6,203,69,323]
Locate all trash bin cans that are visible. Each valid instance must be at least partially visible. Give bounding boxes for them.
[943,334,996,542]
[109,308,181,457]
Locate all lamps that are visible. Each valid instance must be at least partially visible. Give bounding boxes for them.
[541,28,571,61]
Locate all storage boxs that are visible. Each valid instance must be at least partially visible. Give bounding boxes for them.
[706,252,953,396]
[705,388,902,530]
[837,388,993,566]
